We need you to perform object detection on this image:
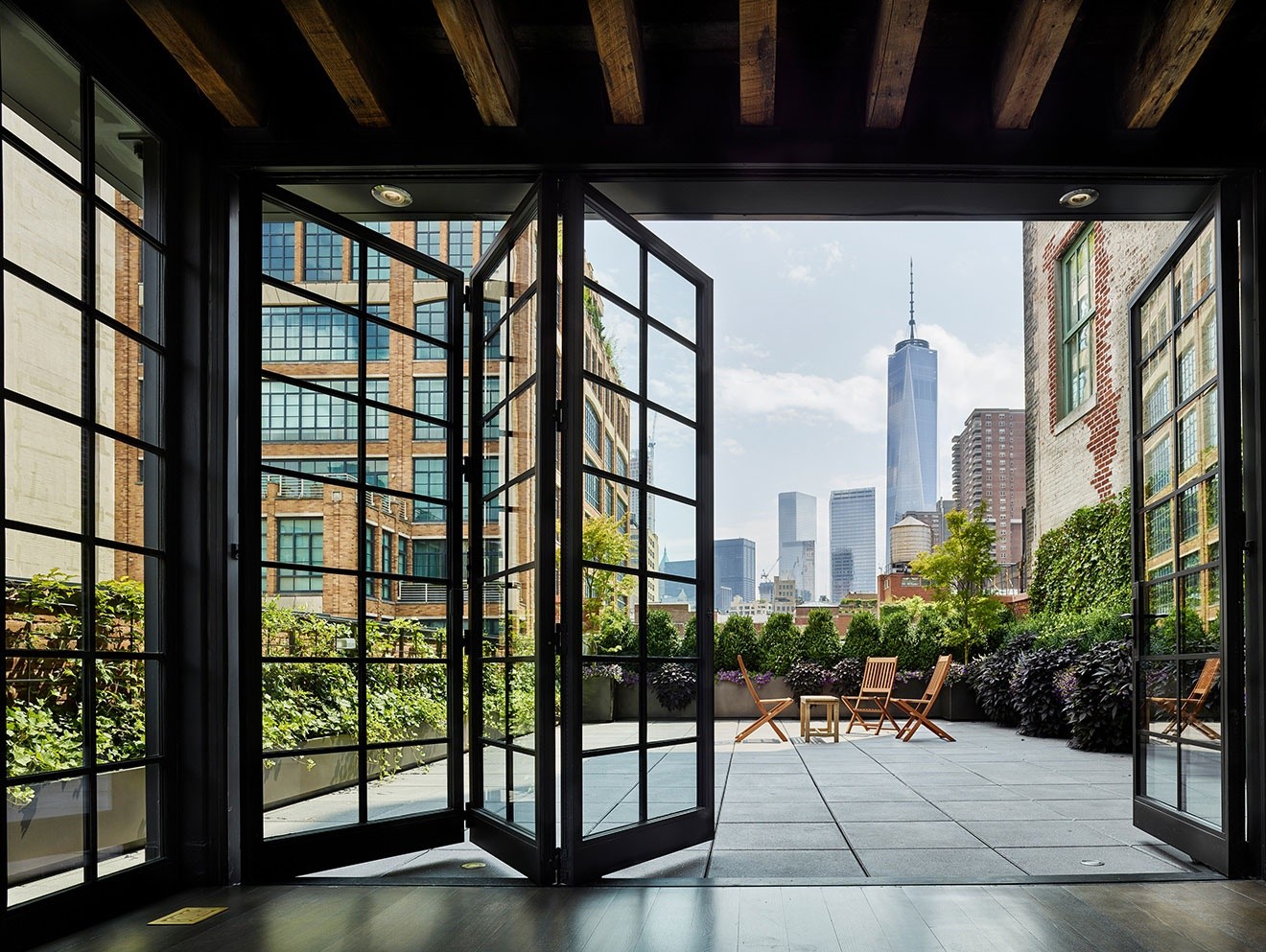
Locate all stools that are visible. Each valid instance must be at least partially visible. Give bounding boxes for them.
[799,694,840,743]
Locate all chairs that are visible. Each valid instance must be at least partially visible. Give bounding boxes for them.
[735,654,794,743]
[889,653,957,743]
[1149,656,1221,744]
[841,656,901,736]
